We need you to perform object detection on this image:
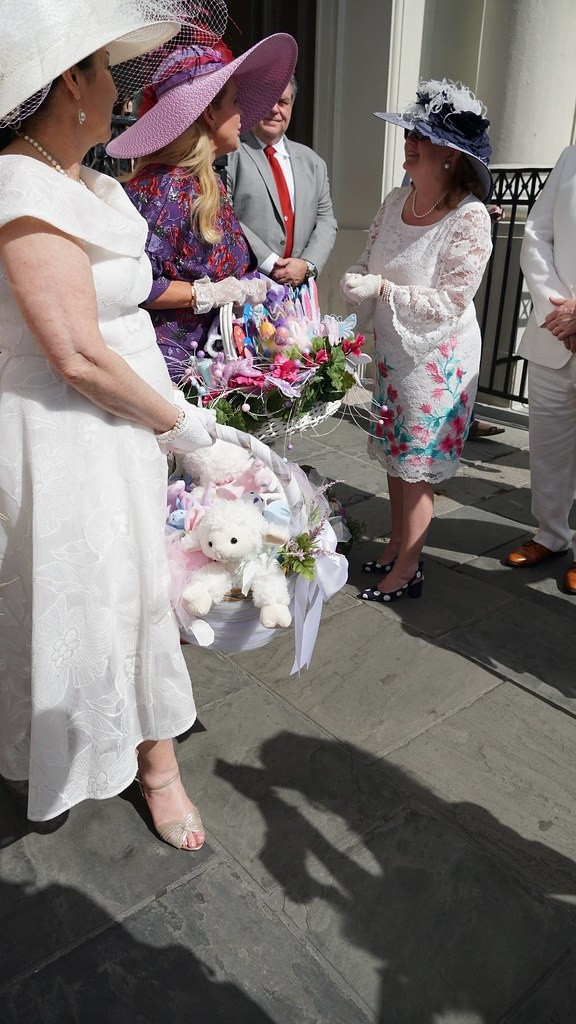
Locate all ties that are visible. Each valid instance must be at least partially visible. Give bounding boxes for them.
[264,145,296,262]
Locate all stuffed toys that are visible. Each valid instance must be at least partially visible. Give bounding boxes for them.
[169,437,292,630]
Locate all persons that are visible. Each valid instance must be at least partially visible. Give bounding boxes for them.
[508,142,575,594]
[0,0,222,853]
[225,68,339,290]
[338,79,505,604]
[108,32,299,401]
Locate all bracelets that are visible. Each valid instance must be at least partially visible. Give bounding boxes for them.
[381,279,390,304]
[191,283,195,308]
[306,261,315,277]
[157,403,183,444]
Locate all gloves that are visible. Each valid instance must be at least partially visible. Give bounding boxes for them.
[340,273,383,305]
[157,389,217,456]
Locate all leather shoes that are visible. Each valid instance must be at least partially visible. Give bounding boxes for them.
[506,540,569,568]
[566,560,576,592]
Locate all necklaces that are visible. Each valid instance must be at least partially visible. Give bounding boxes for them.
[412,190,447,219]
[21,134,91,191]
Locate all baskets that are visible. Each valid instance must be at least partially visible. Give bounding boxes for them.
[166,424,296,645]
[205,300,341,444]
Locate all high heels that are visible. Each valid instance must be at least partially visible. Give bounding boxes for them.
[361,554,398,576]
[361,561,426,602]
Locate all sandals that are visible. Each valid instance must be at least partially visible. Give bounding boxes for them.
[468,420,504,436]
[139,765,204,850]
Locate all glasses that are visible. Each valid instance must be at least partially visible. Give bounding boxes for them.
[404,128,430,141]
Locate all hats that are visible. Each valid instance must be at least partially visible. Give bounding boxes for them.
[107,34,297,158]
[0,0,180,123]
[373,82,494,206]
